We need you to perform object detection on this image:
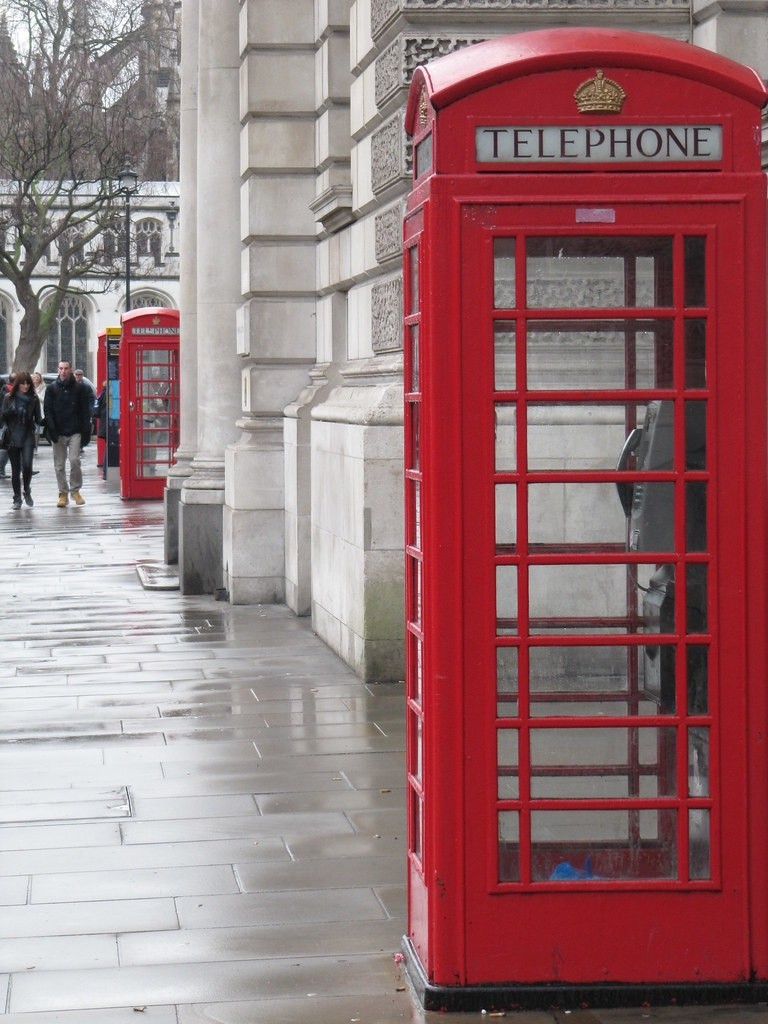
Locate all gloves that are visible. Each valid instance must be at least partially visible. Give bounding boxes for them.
[81,429,91,446]
[47,427,59,443]
[41,418,47,426]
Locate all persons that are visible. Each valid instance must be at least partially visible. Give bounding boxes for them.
[0,369,107,480]
[2,371,49,510]
[44,359,92,508]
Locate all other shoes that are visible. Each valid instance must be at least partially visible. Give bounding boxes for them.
[70,491,85,505]
[0,475,11,478]
[57,492,69,507]
[32,471,40,475]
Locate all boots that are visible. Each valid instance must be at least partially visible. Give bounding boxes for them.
[13,493,22,509]
[21,487,33,507]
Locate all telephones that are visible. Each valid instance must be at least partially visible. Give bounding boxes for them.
[613,398,675,558]
[163,386,170,402]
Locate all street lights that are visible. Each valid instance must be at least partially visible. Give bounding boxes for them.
[118,161,139,313]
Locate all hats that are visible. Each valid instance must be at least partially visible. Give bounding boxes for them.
[75,369,83,375]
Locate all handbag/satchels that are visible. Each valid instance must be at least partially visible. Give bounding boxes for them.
[0,427,10,450]
